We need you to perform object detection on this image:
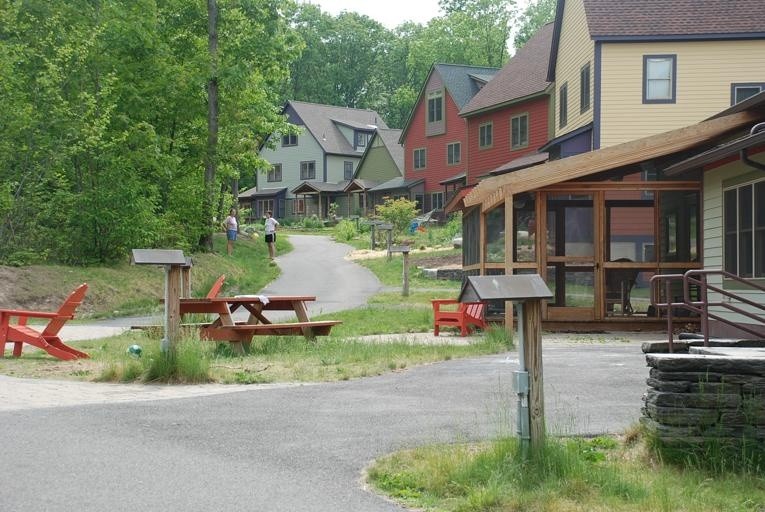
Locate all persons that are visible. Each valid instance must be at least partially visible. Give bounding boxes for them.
[264,210,280,260]
[223,209,237,255]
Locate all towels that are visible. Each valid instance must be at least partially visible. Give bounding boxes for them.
[234,294,270,305]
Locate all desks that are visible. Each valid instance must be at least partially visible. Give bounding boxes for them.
[159,294,320,357]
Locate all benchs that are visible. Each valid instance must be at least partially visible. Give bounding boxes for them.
[130,321,249,338]
[200,320,342,357]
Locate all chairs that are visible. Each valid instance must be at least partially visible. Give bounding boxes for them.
[205,274,227,299]
[430,295,490,337]
[0,281,94,363]
[603,256,642,316]
